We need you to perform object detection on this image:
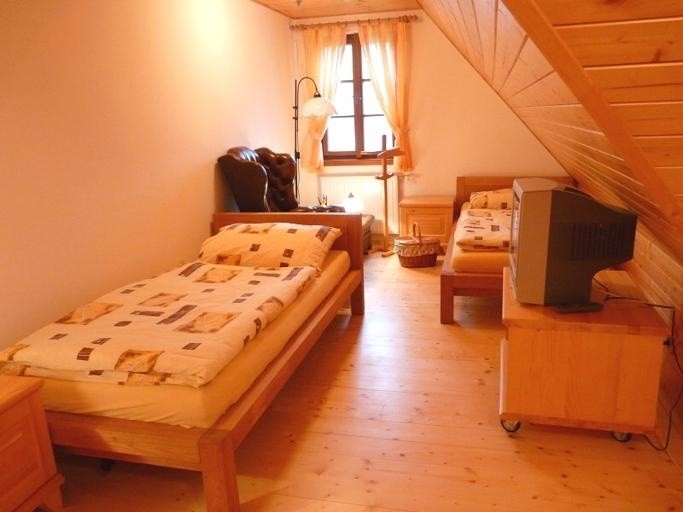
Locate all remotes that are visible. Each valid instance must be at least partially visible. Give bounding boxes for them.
[556,302,603,313]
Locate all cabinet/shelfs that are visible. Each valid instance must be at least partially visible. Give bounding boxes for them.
[500,266,668,443]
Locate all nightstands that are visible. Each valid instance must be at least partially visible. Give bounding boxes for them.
[399,195,453,253]
[0,377,66,512]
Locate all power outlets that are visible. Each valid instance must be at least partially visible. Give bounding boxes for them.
[663,337,674,352]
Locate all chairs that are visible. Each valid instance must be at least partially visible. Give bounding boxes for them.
[218,146,345,214]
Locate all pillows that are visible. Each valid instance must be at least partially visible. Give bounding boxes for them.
[469,189,513,209]
[200,221,343,276]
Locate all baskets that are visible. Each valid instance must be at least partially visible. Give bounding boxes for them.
[394,221,440,268]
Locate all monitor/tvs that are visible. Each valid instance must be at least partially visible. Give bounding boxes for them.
[507,177,637,309]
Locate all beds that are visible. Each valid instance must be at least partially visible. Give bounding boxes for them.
[0,210,364,512]
[441,176,574,324]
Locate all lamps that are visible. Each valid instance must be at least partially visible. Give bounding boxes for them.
[292,73,337,203]
